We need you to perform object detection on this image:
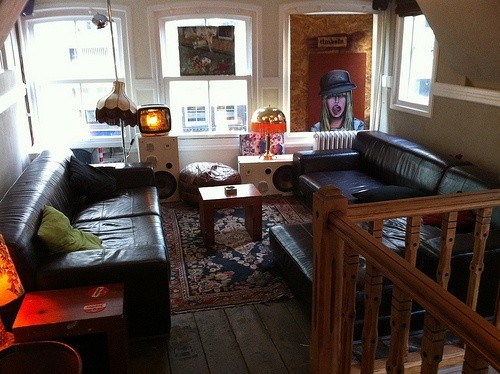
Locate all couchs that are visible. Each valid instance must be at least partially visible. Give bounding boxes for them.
[0,148,171,340]
[269,128,500,341]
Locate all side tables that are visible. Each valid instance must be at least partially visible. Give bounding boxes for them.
[12,282,128,374]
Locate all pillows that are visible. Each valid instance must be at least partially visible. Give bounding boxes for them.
[352,186,422,203]
[422,191,475,225]
[37,204,104,252]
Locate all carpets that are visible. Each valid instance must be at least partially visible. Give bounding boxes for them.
[159,194,315,315]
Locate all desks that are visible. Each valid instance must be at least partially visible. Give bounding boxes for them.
[198,183,263,245]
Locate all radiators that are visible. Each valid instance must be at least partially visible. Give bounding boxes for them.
[313,130,357,150]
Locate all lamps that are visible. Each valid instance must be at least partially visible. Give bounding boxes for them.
[251,104,286,160]
[96,80,138,162]
[0,231,26,347]
